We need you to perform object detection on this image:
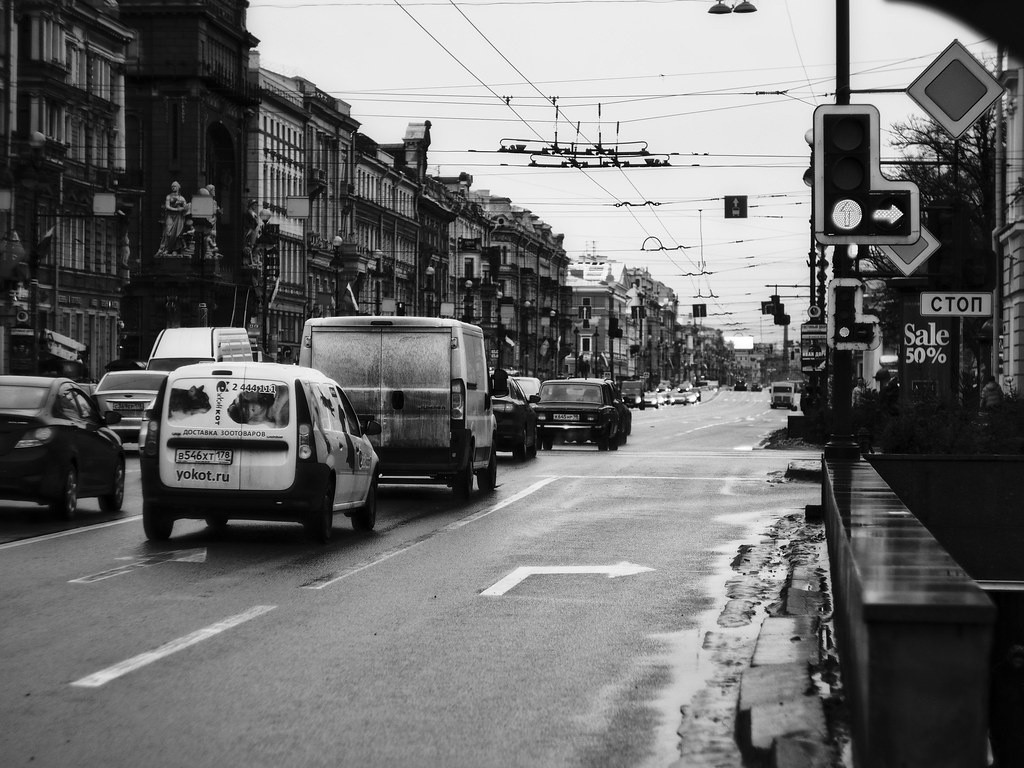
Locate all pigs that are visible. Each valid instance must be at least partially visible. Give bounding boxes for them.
[226,383,288,429]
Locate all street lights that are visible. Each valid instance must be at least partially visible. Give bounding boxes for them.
[427,266,435,317]
[260,209,271,354]
[524,300,530,377]
[465,280,472,323]
[373,248,383,315]
[496,292,503,368]
[333,235,343,316]
[27,131,46,373]
[550,310,556,380]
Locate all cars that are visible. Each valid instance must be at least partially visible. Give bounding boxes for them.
[138,361,382,544]
[0,375,126,520]
[490,371,632,462]
[751,381,763,391]
[644,379,702,409]
[77,370,172,450]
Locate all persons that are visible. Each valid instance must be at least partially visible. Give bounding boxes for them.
[158,181,219,254]
[243,200,264,266]
[980,377,1004,409]
[853,378,866,406]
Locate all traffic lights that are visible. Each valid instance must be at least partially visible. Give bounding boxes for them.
[813,105,921,245]
[760,299,790,325]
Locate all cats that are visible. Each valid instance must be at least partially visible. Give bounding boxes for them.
[168,385,212,421]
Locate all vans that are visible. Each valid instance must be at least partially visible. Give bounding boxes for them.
[145,327,253,371]
[299,316,509,500]
[769,381,795,409]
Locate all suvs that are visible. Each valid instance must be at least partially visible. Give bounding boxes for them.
[734,377,748,391]
[621,381,643,410]
